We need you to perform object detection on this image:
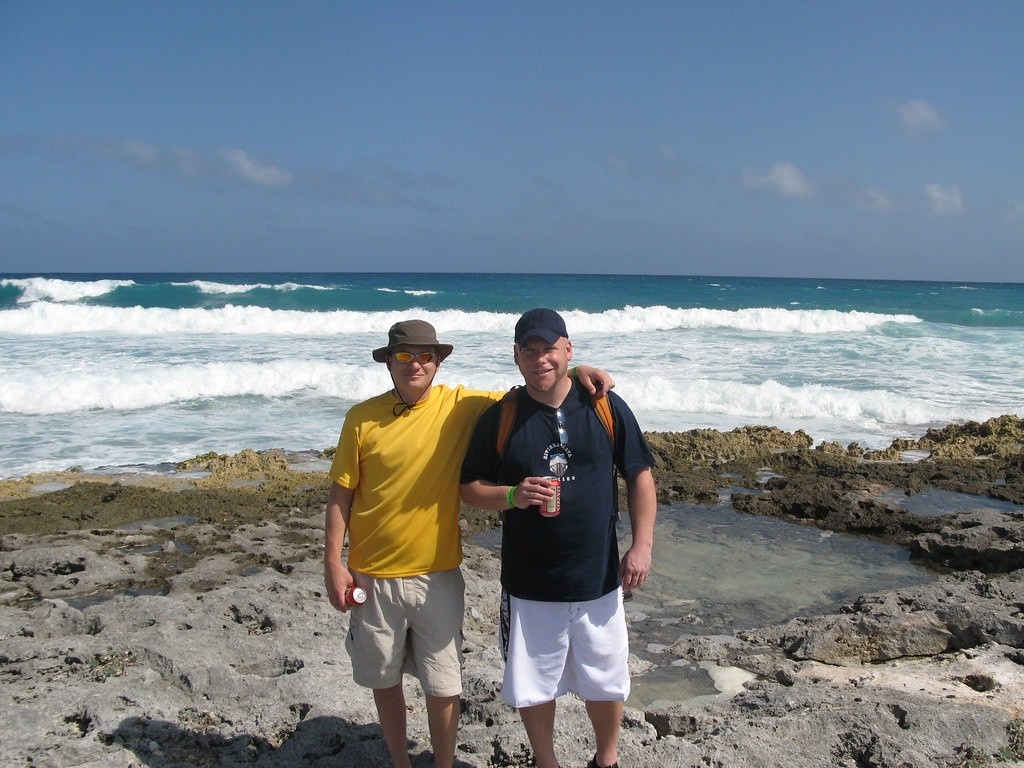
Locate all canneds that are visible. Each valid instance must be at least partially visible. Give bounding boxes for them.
[539,475,560,517]
[345,586,367,605]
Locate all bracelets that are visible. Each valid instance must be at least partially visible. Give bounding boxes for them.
[573,365,578,379]
[507,486,518,508]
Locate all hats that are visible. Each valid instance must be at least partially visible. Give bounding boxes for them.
[514,307,569,351]
[372,320,454,363]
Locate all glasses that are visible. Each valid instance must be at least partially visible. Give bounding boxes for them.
[555,406,569,446]
[390,352,438,364]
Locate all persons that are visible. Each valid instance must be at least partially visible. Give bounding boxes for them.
[324,319,614,768]
[459,308,657,768]
